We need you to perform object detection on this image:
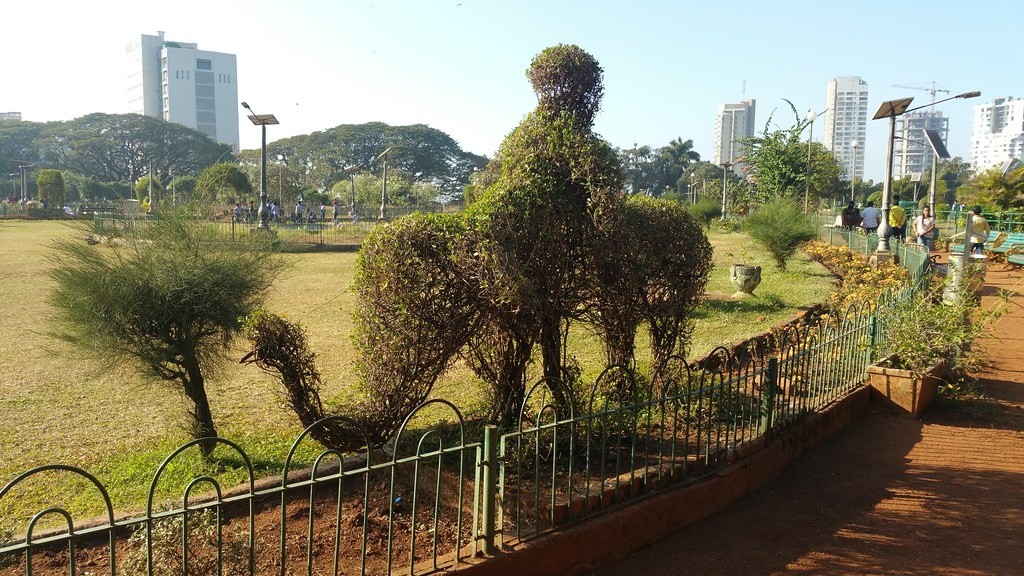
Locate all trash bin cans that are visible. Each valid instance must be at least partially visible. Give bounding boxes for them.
[942,230,990,308]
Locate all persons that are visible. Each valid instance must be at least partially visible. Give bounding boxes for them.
[841,200,858,219]
[859,201,881,232]
[914,207,934,247]
[889,199,906,239]
[970,206,991,254]
[843,205,861,229]
[948,200,967,211]
[890,195,917,243]
[6,192,340,226]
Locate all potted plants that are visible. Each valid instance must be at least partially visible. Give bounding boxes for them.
[860,254,1024,418]
[722,239,768,300]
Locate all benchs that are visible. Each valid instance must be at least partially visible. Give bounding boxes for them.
[947,227,1024,269]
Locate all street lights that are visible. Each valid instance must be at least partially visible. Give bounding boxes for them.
[871,89,984,252]
[380,148,396,219]
[666,185,669,194]
[691,172,694,205]
[241,101,269,230]
[351,162,363,211]
[850,140,859,204]
[687,184,690,202]
[804,111,817,216]
